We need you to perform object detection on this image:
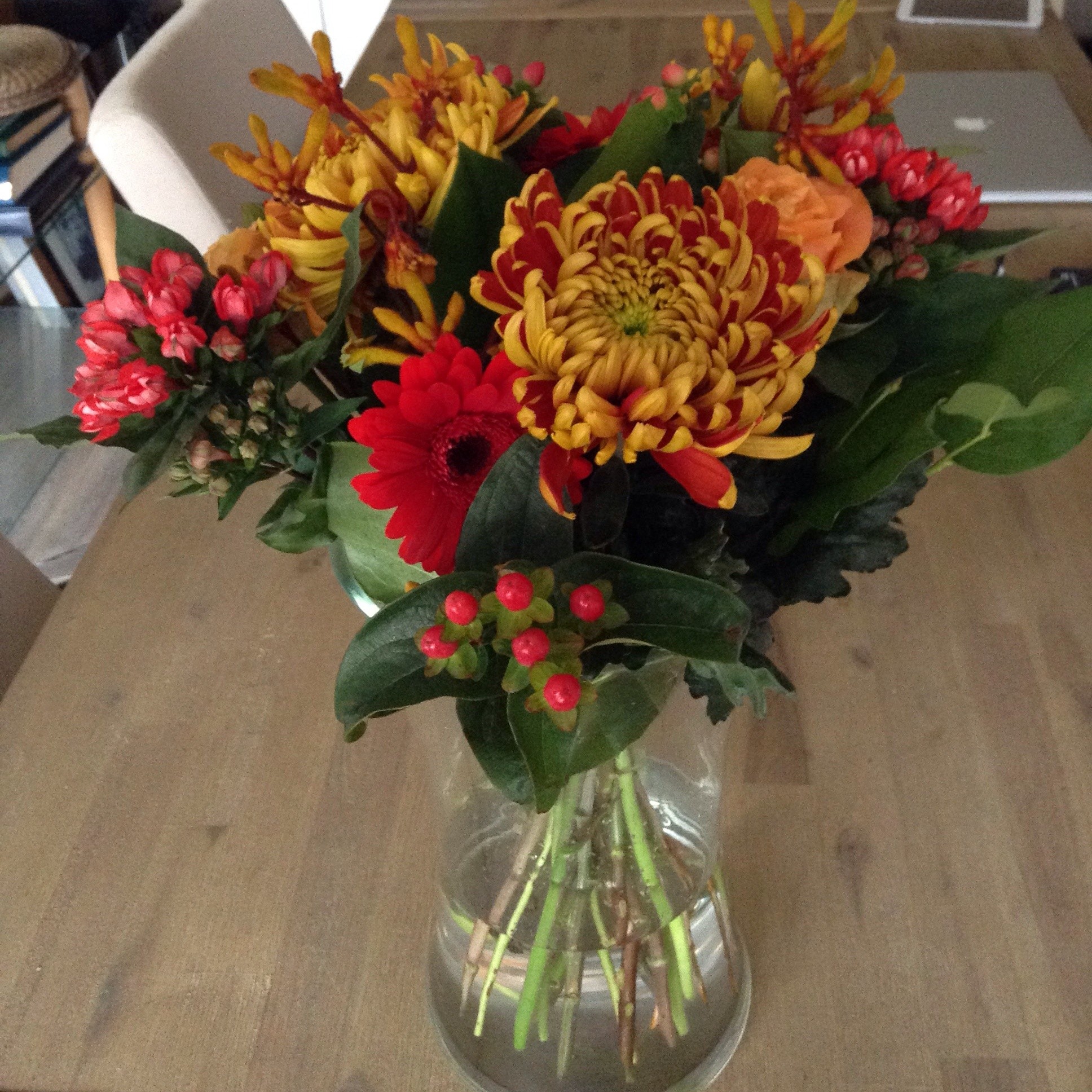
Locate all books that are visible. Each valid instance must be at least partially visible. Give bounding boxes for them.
[0,97,84,240]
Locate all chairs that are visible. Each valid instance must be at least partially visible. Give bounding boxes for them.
[86,0,323,256]
[0,531,61,703]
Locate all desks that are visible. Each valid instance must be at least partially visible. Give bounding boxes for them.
[1,0,1091,1091]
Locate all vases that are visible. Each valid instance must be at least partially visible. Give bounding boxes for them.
[425,676,753,1092]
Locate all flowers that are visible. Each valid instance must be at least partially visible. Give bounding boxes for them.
[1,0,1090,814]
